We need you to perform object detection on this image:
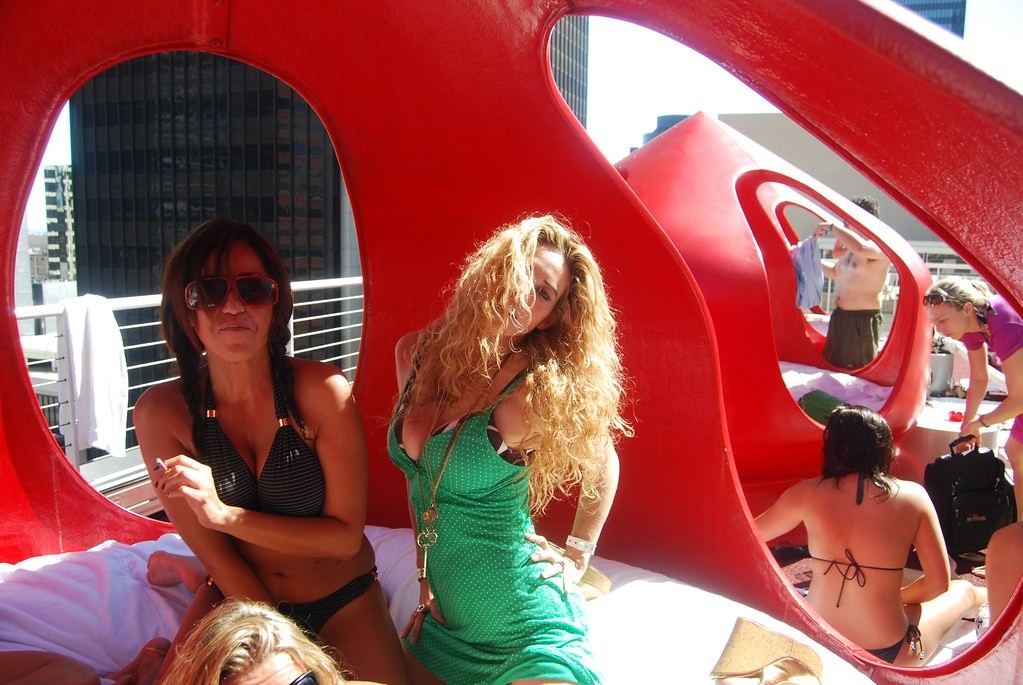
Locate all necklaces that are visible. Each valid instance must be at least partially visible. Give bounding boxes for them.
[416,341,520,580]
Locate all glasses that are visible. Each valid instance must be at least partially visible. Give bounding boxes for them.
[184,276,279,310]
[923,293,972,306]
[289,669,320,685]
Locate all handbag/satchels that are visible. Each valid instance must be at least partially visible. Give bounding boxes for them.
[923,435,1017,554]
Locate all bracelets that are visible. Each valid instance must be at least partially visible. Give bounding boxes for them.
[979,414,990,428]
[829,224,834,232]
[416,567,426,582]
[565,534,597,556]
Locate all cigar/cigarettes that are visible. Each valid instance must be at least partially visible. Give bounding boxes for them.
[154,457,167,472]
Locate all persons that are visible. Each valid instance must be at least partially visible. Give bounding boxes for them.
[752,403,990,670]
[788,195,893,371]
[131,213,414,685]
[101,552,386,685]
[984,523,1023,628]
[385,209,640,685]
[922,277,1023,522]
[0,649,101,685]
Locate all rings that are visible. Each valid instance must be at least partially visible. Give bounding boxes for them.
[416,603,430,617]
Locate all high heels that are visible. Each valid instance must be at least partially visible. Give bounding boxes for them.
[709,617,823,684]
[548,541,611,601]
